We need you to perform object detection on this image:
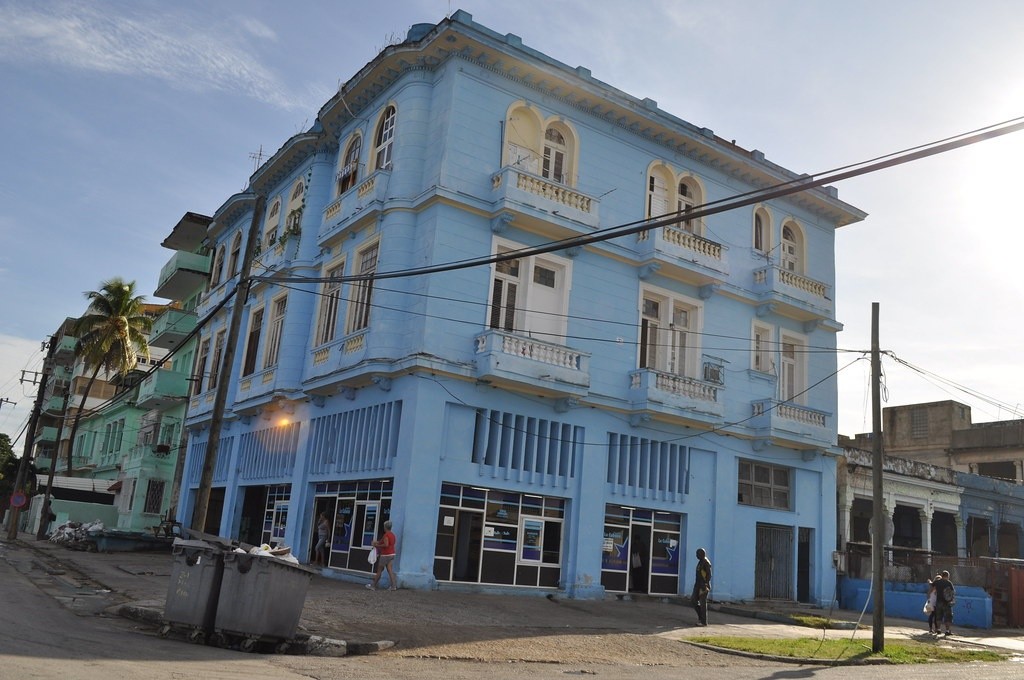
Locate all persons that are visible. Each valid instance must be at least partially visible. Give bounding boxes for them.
[926,570,954,635]
[311,512,330,567]
[38,500,51,540]
[364,521,399,590]
[690,548,712,626]
[21,509,30,532]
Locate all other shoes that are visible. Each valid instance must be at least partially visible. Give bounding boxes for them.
[365,583,376,592]
[311,561,324,571]
[387,585,397,591]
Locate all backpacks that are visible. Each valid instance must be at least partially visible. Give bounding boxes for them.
[943,586,956,606]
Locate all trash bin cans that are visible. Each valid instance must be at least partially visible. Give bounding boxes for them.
[212,550,315,654]
[159,542,225,644]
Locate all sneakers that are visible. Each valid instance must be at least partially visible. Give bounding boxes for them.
[945,630,954,635]
[937,629,941,634]
[929,629,936,634]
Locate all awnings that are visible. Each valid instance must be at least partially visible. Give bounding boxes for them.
[107,481,122,491]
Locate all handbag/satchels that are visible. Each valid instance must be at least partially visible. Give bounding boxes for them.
[922,599,934,616]
[368,546,380,565]
[326,540,331,547]
